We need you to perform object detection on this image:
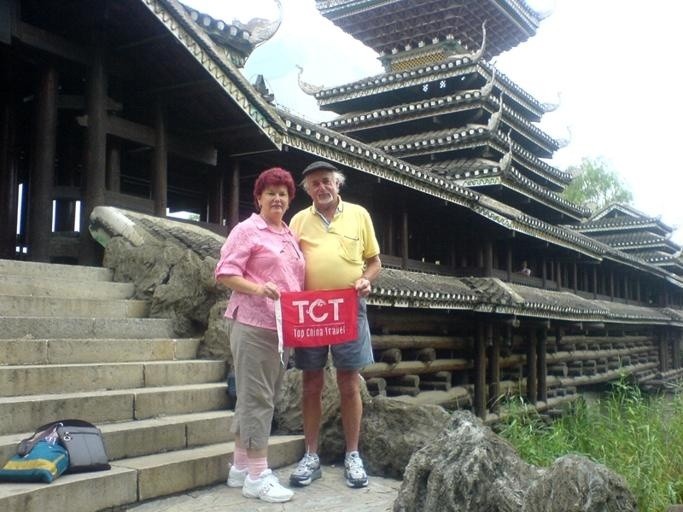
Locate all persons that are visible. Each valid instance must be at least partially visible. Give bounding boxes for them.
[516,260,531,276]
[213,168,306,503]
[287,161,382,487]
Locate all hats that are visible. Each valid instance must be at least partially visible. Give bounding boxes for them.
[301,161,337,175]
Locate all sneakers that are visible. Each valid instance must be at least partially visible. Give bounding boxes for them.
[243,469,293,502]
[227,466,248,487]
[344,450,368,487]
[289,452,322,486]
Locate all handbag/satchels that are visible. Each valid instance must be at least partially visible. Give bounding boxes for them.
[17,419,111,472]
[1,438,70,484]
